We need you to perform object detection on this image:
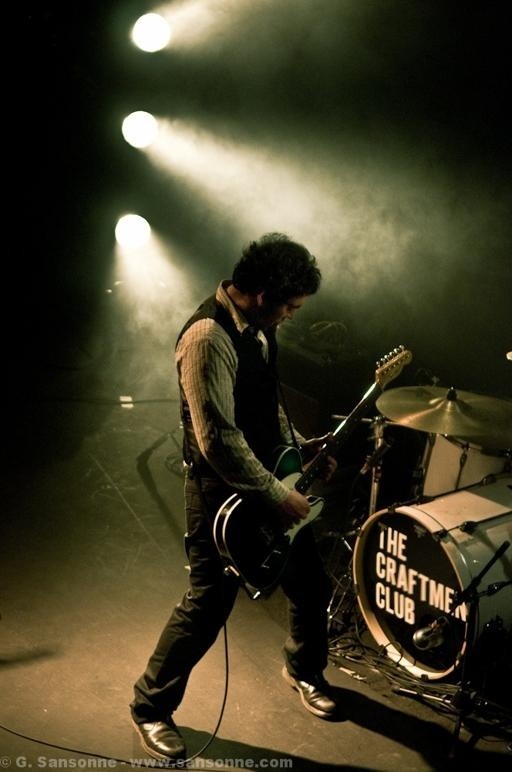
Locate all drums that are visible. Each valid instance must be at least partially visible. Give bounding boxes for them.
[411,431,510,500]
[352,474,512,684]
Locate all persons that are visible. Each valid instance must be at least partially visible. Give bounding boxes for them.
[122,232,349,768]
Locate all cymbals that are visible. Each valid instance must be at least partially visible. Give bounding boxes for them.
[375,385,511,438]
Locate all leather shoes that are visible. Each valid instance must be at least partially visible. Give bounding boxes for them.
[281,665,340,718]
[128,705,187,762]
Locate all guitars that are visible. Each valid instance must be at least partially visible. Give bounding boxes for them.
[212,344,412,591]
